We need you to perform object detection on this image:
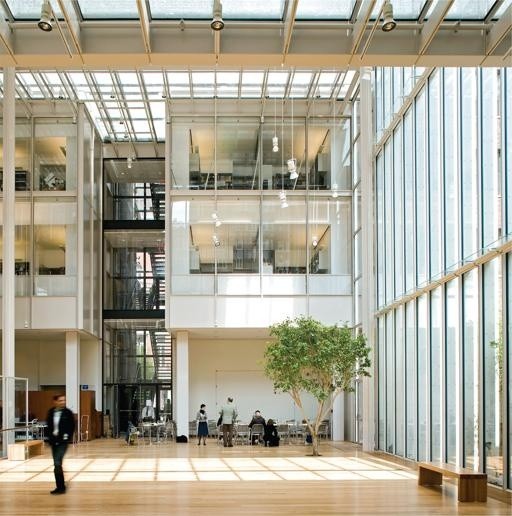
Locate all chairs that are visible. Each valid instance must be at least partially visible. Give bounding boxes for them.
[127,418,330,449]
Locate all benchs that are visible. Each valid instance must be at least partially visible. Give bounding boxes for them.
[417,461,488,502]
[7,439,44,460]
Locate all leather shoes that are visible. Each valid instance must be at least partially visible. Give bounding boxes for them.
[51,487,67,495]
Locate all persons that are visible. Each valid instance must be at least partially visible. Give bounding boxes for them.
[218,397,237,447]
[47,395,75,494]
[248,410,278,447]
[196,404,208,445]
[142,406,154,422]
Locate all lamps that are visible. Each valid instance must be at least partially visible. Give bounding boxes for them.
[272,96,322,246]
[210,13,224,31]
[37,4,53,32]
[162,84,171,125]
[380,1,397,32]
[210,213,222,248]
[127,142,133,169]
[110,85,128,139]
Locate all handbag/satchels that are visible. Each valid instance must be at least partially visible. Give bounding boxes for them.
[176,435,187,443]
[217,415,222,426]
